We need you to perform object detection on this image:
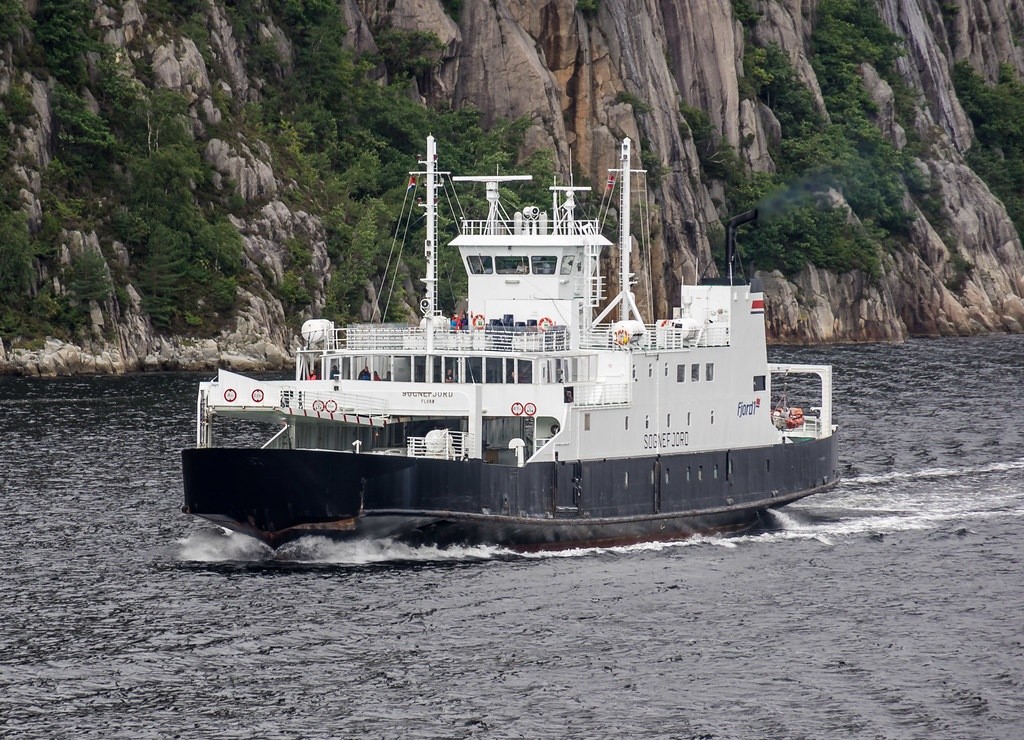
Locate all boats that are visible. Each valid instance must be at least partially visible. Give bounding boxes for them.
[178,134,850,570]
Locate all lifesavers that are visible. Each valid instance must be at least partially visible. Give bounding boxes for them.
[616,330,631,346]
[539,317,553,332]
[472,314,486,331]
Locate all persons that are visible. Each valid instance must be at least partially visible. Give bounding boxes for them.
[358,366,391,381]
[510,369,531,382]
[446,365,482,383]
[515,256,556,274]
[557,368,564,383]
[308,362,339,380]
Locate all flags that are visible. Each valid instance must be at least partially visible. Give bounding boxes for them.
[407,176,416,194]
[607,174,615,190]
